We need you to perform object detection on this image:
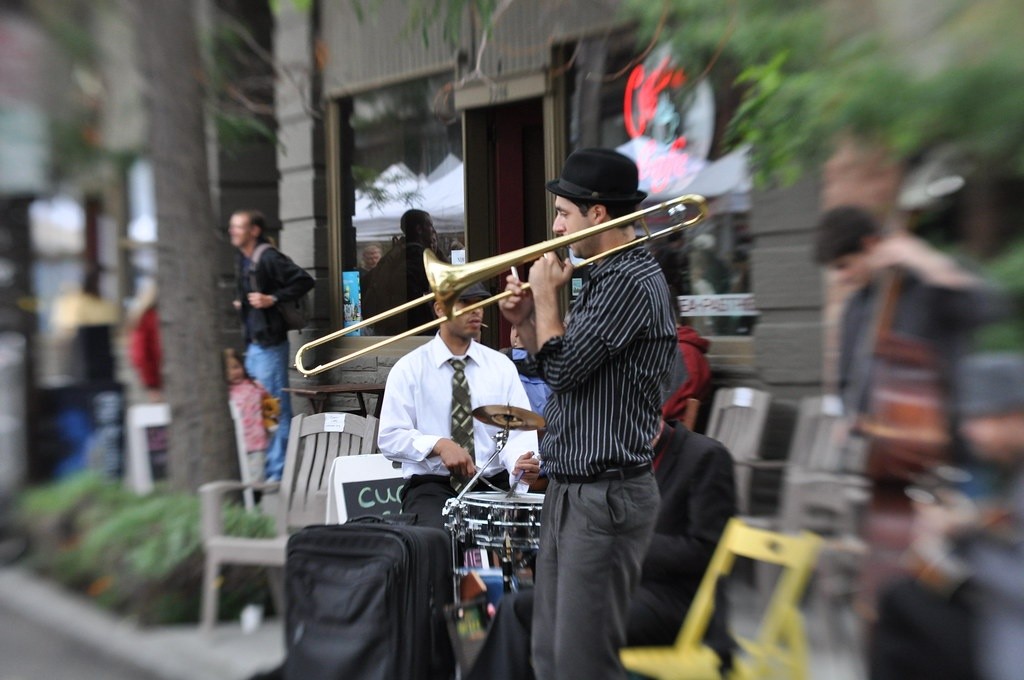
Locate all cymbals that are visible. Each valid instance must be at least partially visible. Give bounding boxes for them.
[469,406,548,430]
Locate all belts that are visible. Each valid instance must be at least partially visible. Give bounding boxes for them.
[553,462,649,483]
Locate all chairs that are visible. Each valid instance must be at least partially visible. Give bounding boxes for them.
[619,518,827,680]
[734,397,866,637]
[199,412,379,628]
[704,387,768,511]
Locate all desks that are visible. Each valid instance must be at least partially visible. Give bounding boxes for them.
[281,383,385,421]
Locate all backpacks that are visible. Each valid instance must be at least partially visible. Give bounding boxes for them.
[249,243,313,330]
[360,236,425,330]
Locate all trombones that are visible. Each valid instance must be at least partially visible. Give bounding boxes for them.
[293,194,711,376]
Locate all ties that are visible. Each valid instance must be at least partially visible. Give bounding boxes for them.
[448,355,478,495]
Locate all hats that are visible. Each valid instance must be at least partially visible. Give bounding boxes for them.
[428,280,492,306]
[944,349,1024,415]
[546,148,648,205]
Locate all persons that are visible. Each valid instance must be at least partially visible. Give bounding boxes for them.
[375,281,542,624]
[497,149,677,680]
[360,208,464,335]
[223,209,315,492]
[465,410,759,679]
[801,146,1024,680]
[128,299,167,405]
[504,304,715,453]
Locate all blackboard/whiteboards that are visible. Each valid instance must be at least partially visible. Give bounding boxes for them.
[127,405,173,493]
[325,452,409,525]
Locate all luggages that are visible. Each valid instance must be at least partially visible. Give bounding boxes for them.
[283,513,457,680]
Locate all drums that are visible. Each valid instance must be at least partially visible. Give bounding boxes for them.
[447,492,552,550]
